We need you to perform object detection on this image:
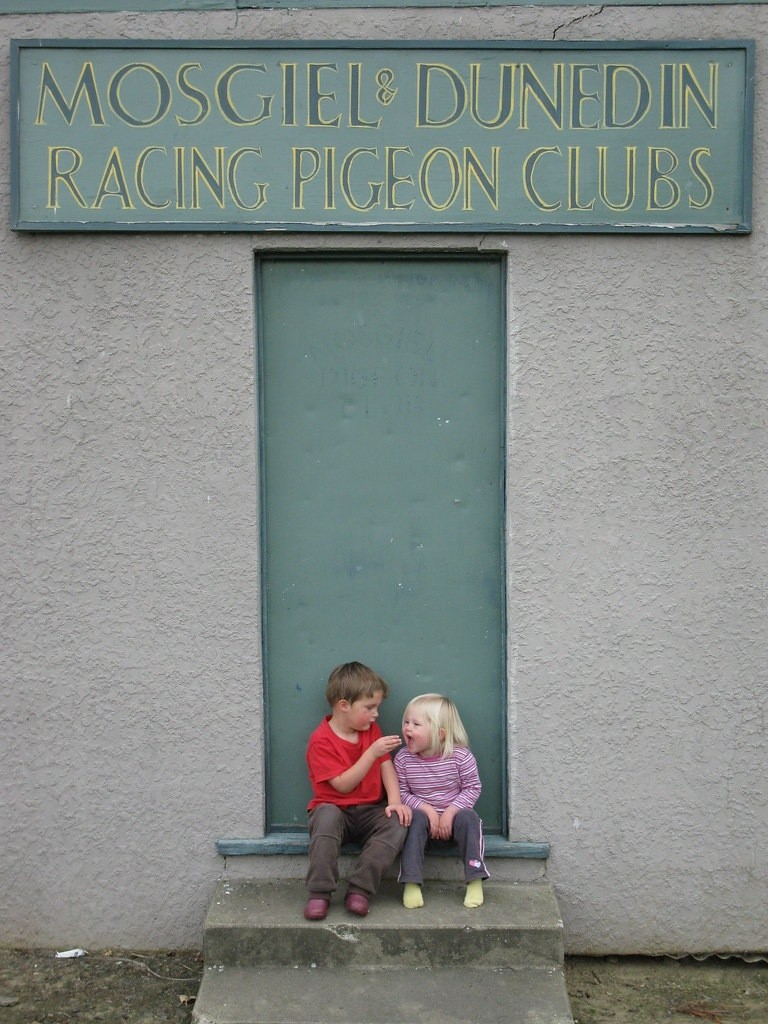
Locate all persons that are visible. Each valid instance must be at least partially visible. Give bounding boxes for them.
[391,691,492,909]
[304,661,415,921]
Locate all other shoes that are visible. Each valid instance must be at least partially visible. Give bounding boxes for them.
[305,895,327,919]
[345,891,371,917]
